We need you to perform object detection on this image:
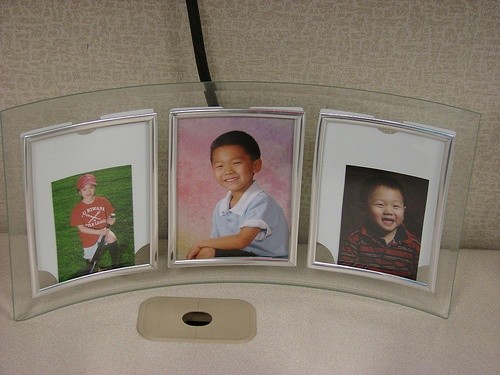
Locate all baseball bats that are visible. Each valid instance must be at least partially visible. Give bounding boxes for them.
[86,213,117,273]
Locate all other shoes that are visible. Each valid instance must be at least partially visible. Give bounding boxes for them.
[113,261,124,268]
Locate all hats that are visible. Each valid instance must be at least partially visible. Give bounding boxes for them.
[77,174,98,191]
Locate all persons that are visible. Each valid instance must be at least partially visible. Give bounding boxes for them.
[71,174,123,274]
[186,130,291,262]
[338,174,422,282]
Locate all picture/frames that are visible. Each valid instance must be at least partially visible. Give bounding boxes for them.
[167,106,306,269]
[19,108,160,299]
[307,108,455,297]
